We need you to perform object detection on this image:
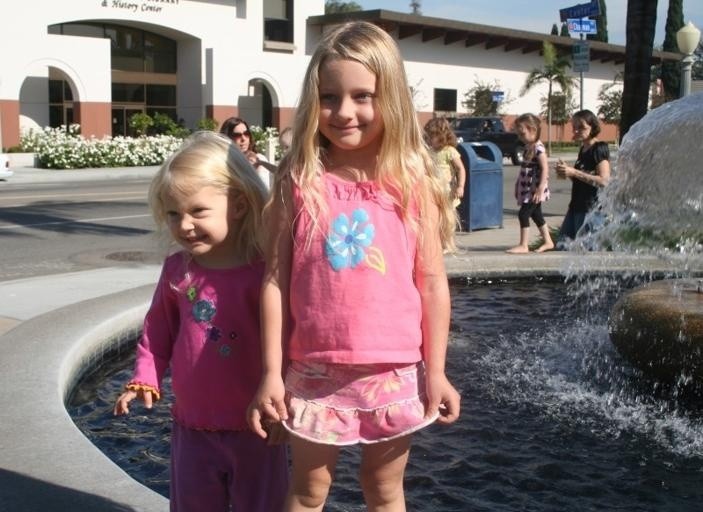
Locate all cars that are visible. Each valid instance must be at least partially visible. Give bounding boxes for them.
[0,153,14,182]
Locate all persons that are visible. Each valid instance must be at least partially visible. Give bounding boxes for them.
[114,129,290,512]
[554,110,610,247]
[245,19,462,511]
[505,113,554,255]
[220,116,270,195]
[254,127,293,181]
[424,116,466,235]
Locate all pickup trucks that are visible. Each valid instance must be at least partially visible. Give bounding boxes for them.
[450,116,525,165]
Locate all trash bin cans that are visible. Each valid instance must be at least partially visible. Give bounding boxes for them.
[458,141,502,232]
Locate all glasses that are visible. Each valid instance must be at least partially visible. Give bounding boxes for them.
[231,131,251,139]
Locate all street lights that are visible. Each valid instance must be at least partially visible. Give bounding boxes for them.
[676,21,701,97]
